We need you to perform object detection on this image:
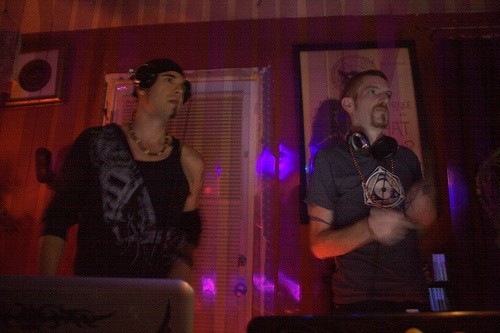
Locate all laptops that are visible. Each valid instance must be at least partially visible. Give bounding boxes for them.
[0,274,195,333]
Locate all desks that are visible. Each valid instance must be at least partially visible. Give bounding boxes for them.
[249,309,500,333]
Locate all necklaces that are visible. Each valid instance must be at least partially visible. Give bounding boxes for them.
[128,121,169,156]
[349,131,395,210]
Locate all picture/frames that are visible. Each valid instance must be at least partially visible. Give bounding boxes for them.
[3,44,66,106]
[292,39,432,227]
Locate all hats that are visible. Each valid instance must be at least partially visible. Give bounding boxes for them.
[146,59,184,76]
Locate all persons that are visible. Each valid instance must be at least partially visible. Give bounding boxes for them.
[41,58,203,280]
[306,70,439,314]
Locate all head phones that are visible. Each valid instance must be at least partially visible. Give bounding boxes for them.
[345,130,399,160]
